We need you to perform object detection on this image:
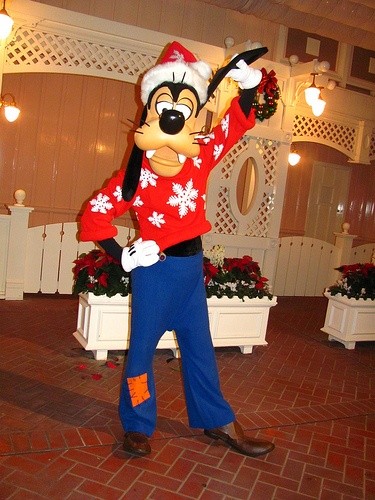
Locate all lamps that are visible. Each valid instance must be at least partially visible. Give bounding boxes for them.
[0,0,14,40]
[288,143,301,166]
[305,73,320,105]
[0,93,21,123]
[312,86,327,117]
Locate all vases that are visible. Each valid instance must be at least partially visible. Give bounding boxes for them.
[72,292,278,360]
[320,288,375,350]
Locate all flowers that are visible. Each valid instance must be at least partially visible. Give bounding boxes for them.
[326,263,375,301]
[72,249,274,302]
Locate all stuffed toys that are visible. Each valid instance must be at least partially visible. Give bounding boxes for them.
[76,40,275,455]
